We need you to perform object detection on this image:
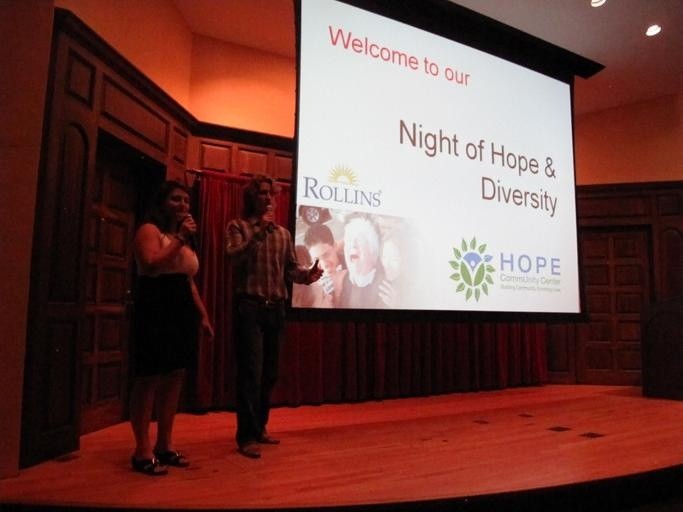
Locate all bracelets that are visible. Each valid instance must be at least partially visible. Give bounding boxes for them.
[174,234,185,246]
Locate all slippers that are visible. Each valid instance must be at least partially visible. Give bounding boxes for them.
[153,449,189,466]
[131,456,168,474]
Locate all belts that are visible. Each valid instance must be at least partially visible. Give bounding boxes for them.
[239,294,286,305]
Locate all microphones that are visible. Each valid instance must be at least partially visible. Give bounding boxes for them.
[264,205,273,233]
[183,213,198,250]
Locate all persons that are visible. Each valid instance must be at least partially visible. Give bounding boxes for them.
[337,211,402,309]
[304,223,347,296]
[127,178,215,476]
[292,244,334,309]
[222,173,324,459]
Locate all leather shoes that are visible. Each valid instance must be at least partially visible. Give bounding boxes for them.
[262,433,280,444]
[239,443,261,456]
